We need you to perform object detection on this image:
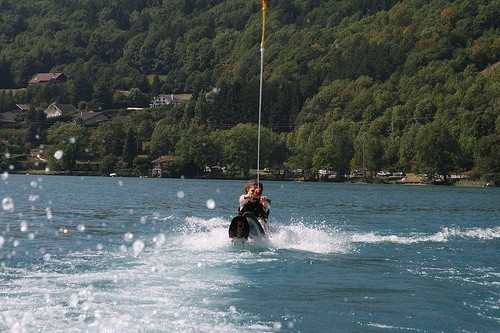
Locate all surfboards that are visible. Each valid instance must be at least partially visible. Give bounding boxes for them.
[226,211,266,238]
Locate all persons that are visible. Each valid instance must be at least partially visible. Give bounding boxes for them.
[239,179,270,224]
